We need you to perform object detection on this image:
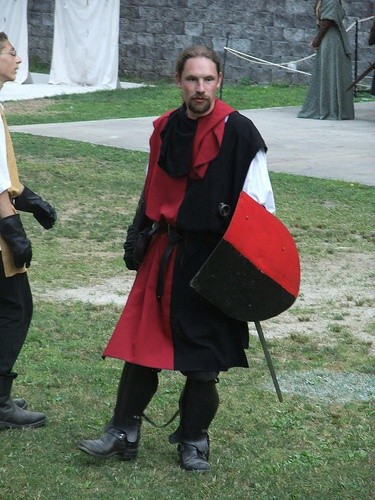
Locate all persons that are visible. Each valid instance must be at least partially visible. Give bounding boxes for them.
[0,32,57,430]
[297,0,355,118]
[76,45,277,470]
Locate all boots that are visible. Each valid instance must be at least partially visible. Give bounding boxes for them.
[0,371,46,428]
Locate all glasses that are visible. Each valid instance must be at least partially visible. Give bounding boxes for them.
[0,50,16,56]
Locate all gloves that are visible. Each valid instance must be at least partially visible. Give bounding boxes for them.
[14,186,57,229]
[0,214,32,268]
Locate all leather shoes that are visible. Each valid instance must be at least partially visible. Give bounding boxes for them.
[77,431,141,460]
[176,438,212,472]
